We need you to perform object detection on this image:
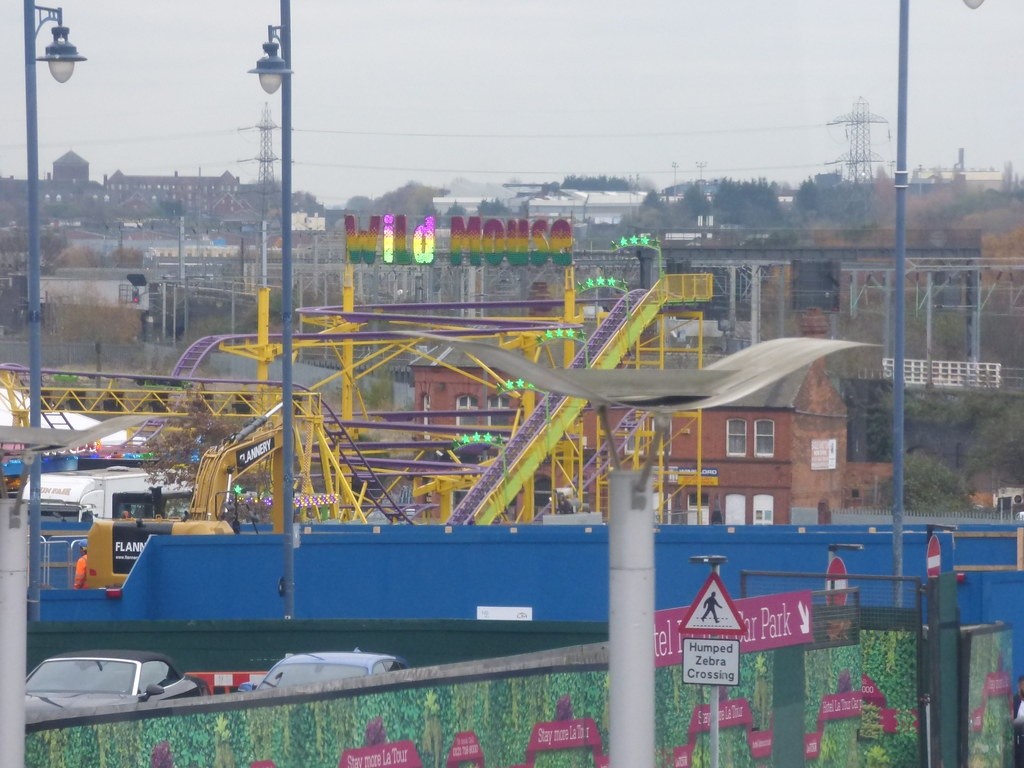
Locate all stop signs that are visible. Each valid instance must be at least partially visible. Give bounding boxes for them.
[925,534,943,578]
[825,556,847,608]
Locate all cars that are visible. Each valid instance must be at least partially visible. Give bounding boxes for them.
[237,649,411,697]
[23,649,210,710]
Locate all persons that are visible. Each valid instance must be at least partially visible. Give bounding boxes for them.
[73,539,88,591]
[120,504,131,519]
[154,511,165,519]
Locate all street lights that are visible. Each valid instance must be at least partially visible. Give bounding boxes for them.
[19,0,90,621]
[253,0,294,621]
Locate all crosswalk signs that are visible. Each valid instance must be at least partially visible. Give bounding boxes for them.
[677,571,747,635]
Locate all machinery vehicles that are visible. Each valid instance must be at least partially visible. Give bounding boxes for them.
[72,386,322,595]
